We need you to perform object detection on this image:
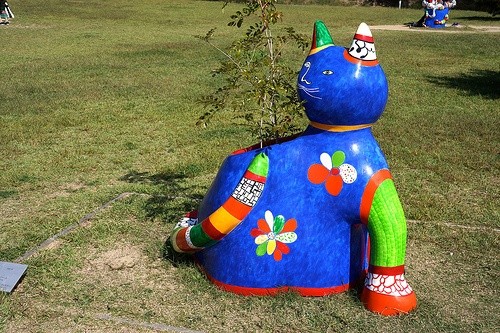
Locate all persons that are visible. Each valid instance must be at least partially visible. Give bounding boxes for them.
[0,0,9,25]
